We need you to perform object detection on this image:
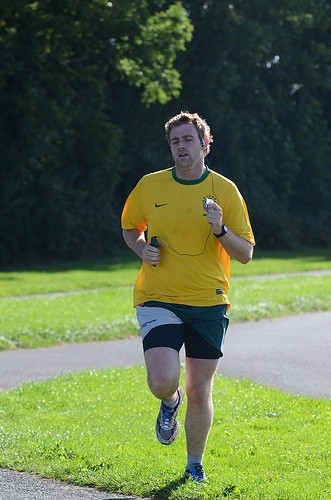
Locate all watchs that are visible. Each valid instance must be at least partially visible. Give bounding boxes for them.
[212,224,228,239]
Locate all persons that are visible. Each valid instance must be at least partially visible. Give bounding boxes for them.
[121,112,256,489]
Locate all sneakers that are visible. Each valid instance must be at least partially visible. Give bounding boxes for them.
[185,464,206,484]
[155,386,184,445]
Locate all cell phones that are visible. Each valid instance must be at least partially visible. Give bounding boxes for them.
[150,237,158,267]
